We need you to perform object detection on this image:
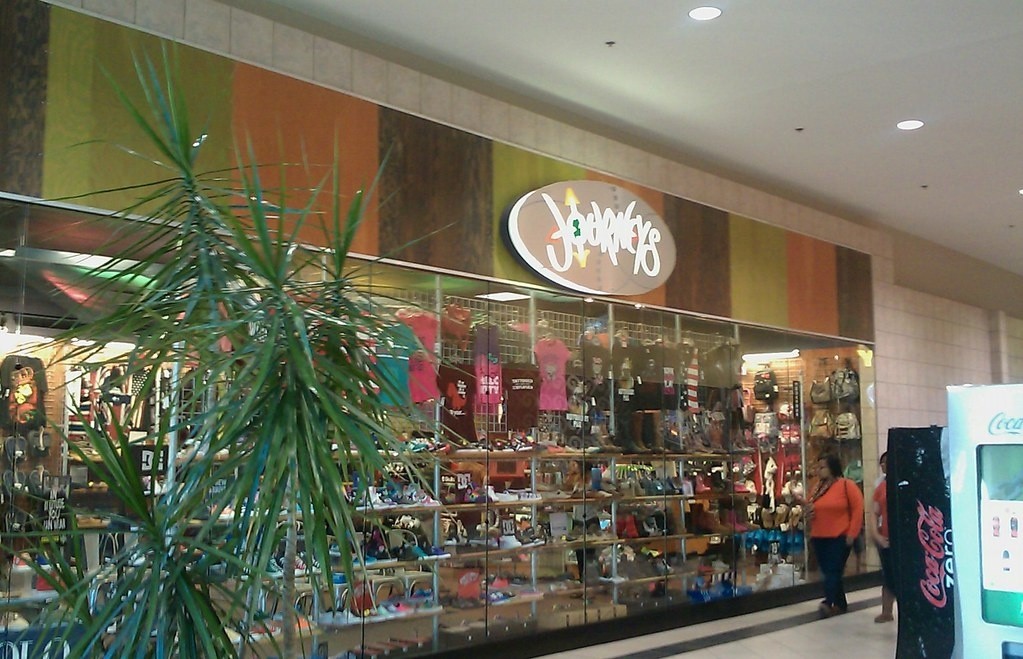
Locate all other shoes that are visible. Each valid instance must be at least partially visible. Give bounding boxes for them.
[0,389,804,635]
[819,602,848,618]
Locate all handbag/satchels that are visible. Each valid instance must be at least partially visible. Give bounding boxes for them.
[854,530,866,553]
[740,389,754,423]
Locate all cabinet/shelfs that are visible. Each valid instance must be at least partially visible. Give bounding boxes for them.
[66,449,749,649]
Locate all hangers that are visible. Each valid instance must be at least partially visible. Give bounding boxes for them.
[685,331,695,346]
[643,330,653,343]
[614,323,630,339]
[538,311,549,327]
[473,310,506,336]
[405,297,423,312]
[543,329,556,341]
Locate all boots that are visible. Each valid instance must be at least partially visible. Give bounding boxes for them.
[874,588,895,623]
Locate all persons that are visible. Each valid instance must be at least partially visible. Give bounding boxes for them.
[867,451,897,622]
[806,453,864,617]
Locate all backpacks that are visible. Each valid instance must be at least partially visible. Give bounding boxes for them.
[807,364,862,446]
[843,458,864,483]
[752,363,779,401]
